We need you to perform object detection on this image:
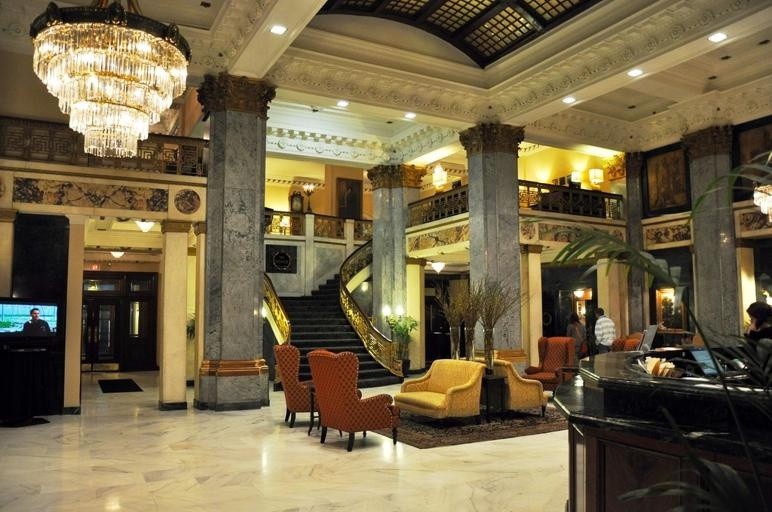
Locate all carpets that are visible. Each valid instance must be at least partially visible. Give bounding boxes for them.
[368,407,571,449]
[99,378,144,393]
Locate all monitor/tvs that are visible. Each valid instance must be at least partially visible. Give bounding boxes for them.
[0,297,66,352]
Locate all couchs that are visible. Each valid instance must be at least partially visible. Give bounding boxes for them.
[394,359,484,428]
[522,336,577,393]
[466,356,547,417]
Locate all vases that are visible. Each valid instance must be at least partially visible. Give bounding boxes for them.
[448,327,461,360]
[464,328,475,361]
[481,328,494,374]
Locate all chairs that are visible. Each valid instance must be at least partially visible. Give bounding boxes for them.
[272,344,327,428]
[307,350,400,451]
[614,332,642,351]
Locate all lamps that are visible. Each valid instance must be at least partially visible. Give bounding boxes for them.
[28,0,191,158]
[303,184,316,213]
[431,163,449,192]
[753,185,772,223]
[570,168,608,190]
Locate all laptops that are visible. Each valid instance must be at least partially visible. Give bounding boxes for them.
[688,346,747,379]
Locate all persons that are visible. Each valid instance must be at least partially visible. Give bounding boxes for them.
[593,308,616,354]
[25,308,50,337]
[744,302,772,390]
[567,312,586,375]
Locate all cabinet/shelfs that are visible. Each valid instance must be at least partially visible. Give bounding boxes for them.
[654,329,695,347]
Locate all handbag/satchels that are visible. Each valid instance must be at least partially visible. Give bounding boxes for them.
[581,343,588,353]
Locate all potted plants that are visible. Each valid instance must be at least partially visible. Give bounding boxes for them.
[385,315,418,378]
